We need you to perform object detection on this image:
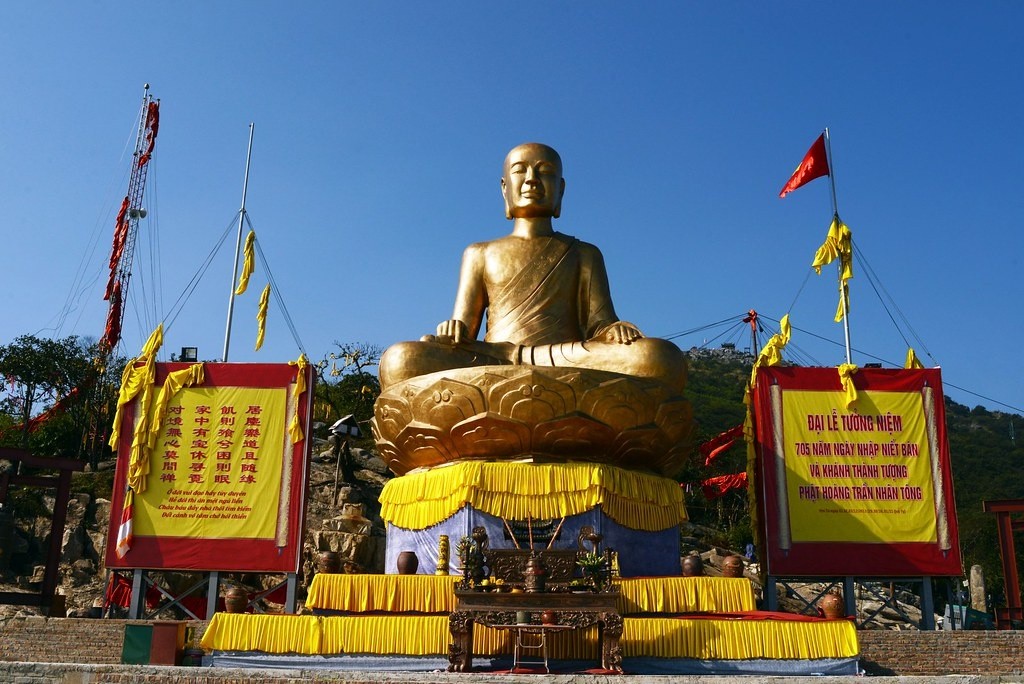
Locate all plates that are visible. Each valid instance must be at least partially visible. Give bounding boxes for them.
[492,584,512,590]
[474,584,489,589]
[566,585,594,594]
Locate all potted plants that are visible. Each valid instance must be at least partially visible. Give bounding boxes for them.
[181,623,206,666]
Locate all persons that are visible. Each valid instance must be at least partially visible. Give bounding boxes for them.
[377,142,686,395]
[745,536,757,562]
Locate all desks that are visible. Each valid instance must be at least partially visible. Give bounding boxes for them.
[491,624,571,672]
[148,621,187,667]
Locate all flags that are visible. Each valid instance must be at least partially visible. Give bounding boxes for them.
[777,131,831,200]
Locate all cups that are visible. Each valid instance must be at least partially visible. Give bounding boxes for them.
[515,611,532,624]
